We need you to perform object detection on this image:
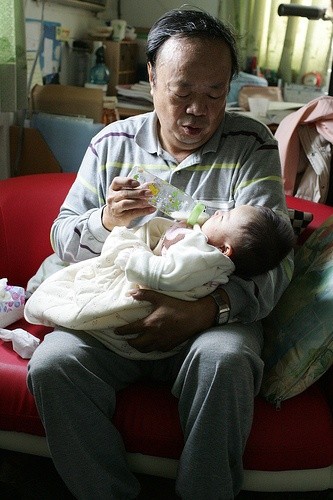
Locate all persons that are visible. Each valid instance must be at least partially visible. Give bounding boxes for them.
[26,4,295,500]
[24,205,297,359]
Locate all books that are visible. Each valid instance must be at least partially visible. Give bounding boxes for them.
[115,80,154,109]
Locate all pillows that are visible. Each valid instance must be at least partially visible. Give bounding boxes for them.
[261,216,333,406]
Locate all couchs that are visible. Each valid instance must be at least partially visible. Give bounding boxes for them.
[0,173,333,493]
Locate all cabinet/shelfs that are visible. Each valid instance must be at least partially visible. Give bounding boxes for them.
[103,40,140,96]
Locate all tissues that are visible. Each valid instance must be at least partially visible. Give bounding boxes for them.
[0,277,26,329]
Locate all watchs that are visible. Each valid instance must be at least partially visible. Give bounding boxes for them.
[211,291,230,325]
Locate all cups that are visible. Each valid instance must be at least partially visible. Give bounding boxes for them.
[111,19,127,42]
[247,98,267,117]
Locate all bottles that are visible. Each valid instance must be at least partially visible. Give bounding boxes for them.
[121,163,210,226]
